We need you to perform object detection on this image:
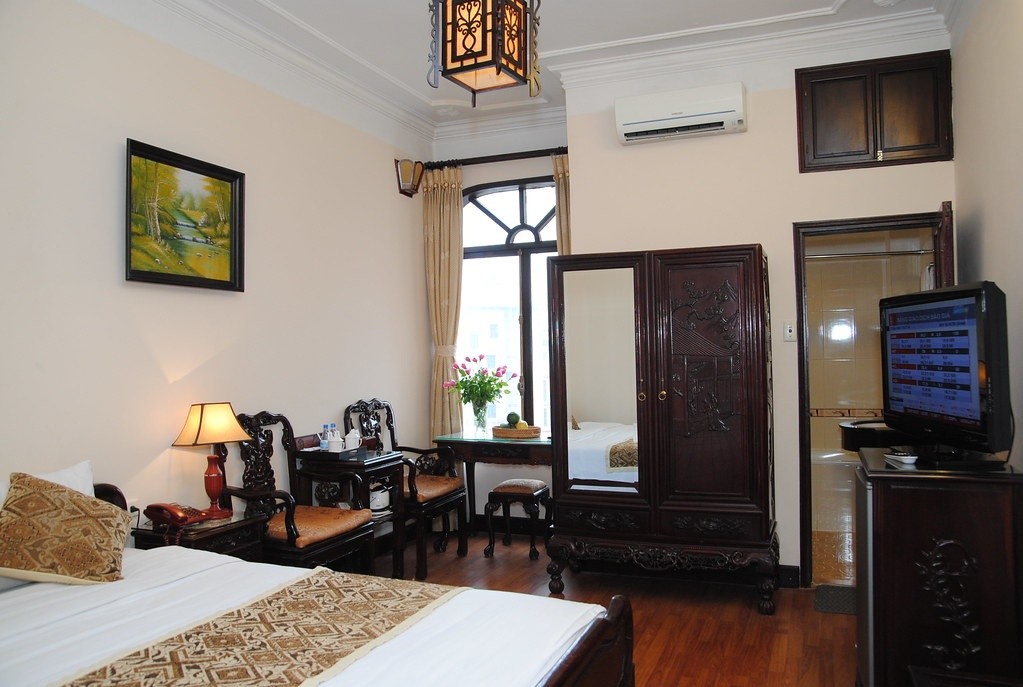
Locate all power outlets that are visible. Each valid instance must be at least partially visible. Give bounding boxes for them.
[126,499,141,517]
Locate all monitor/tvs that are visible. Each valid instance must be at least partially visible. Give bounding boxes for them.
[879,281,1013,468]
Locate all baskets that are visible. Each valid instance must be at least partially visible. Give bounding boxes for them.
[492,425,540,438]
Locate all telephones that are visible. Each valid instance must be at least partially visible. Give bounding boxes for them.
[143,502,209,527]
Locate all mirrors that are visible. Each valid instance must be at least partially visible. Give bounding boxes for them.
[560,268,641,493]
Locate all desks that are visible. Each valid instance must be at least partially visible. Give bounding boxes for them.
[432,428,552,549]
[305,450,405,580]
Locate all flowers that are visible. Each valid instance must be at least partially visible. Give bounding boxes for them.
[440,355,516,401]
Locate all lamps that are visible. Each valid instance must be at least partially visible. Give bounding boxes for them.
[173,401,253,519]
[394,157,425,198]
[427,0,544,108]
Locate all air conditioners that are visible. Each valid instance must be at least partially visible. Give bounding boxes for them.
[614,79,747,146]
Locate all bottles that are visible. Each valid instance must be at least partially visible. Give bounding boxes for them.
[319,423,340,450]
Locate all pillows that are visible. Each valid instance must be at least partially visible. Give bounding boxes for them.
[0,471,131,584]
[0,460,95,500]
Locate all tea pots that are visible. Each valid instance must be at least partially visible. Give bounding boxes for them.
[369,484,394,510]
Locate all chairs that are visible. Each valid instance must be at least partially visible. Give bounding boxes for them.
[345,398,467,583]
[214,410,374,574]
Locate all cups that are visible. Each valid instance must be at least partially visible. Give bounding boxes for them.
[345,429,362,453]
[328,438,344,452]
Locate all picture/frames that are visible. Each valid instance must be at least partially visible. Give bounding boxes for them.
[126,137,246,295]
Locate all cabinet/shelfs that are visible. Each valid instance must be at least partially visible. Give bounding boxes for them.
[795,49,957,174]
[545,244,782,614]
[851,449,1023,686]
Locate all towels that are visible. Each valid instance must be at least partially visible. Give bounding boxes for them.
[919,264,934,292]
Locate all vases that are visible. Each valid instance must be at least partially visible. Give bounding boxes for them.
[469,398,486,439]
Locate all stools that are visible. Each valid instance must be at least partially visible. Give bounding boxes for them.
[481,478,551,561]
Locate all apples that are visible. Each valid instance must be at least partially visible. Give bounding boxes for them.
[516,421,528,429]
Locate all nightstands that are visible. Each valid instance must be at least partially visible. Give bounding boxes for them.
[128,520,264,564]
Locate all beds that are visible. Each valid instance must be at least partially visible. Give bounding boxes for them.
[0,482,636,687]
[567,420,638,481]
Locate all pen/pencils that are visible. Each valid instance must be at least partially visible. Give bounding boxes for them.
[317,433,321,440]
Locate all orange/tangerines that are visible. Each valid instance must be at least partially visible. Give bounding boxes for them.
[507,412,519,424]
